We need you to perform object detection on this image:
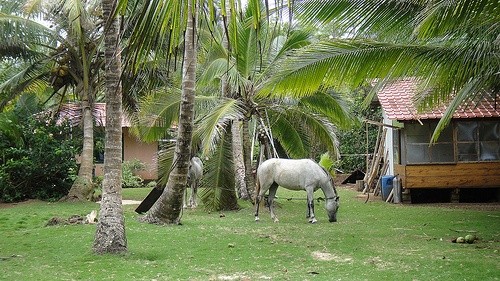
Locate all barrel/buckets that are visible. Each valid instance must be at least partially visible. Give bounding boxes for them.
[381,175,394,201]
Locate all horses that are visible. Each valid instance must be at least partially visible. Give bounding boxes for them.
[182,156,203,209]
[254,158,340,224]
